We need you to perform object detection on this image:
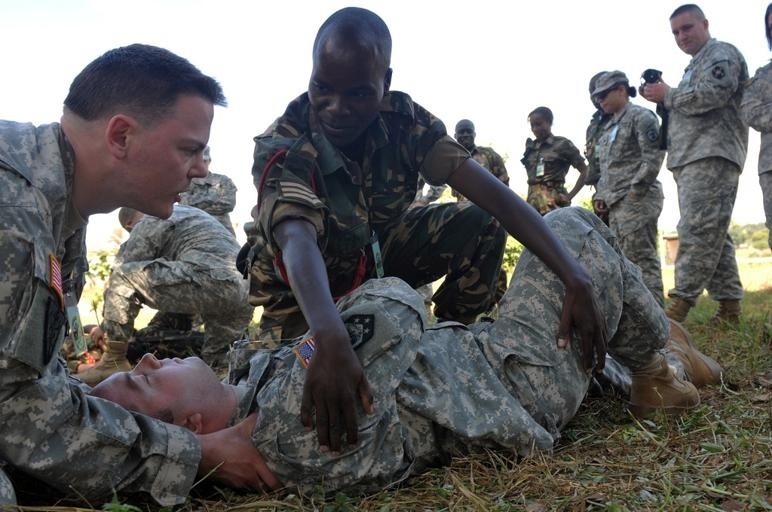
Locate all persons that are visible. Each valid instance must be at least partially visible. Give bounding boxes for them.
[87,206,723,496]
[452,120,509,313]
[522,107,587,216]
[237,7,607,454]
[592,70,665,308]
[0,45,282,512]
[586,73,612,226]
[72,205,256,388]
[410,172,447,307]
[178,145,235,237]
[645,5,749,331]
[741,4,772,252]
[63,311,250,367]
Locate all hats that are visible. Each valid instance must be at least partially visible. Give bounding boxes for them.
[202,146,209,161]
[591,70,628,96]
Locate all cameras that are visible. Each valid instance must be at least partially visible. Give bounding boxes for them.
[641,69,662,83]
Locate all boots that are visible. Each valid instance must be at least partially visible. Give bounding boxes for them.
[703,300,740,328]
[71,339,134,387]
[628,354,700,419]
[664,317,726,388]
[663,298,690,322]
